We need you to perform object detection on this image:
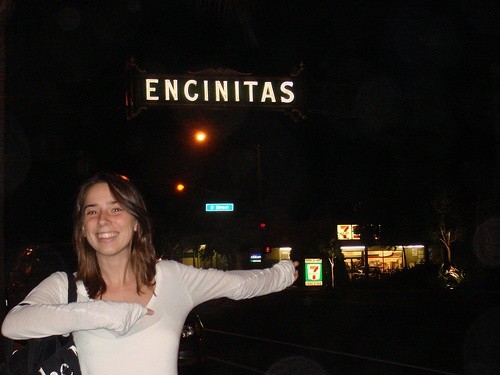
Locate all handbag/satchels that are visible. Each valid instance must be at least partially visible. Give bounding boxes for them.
[9,271,81,375]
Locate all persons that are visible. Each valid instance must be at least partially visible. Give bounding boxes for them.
[2,173,299,374]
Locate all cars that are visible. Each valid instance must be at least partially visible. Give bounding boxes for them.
[178,309,209,370]
